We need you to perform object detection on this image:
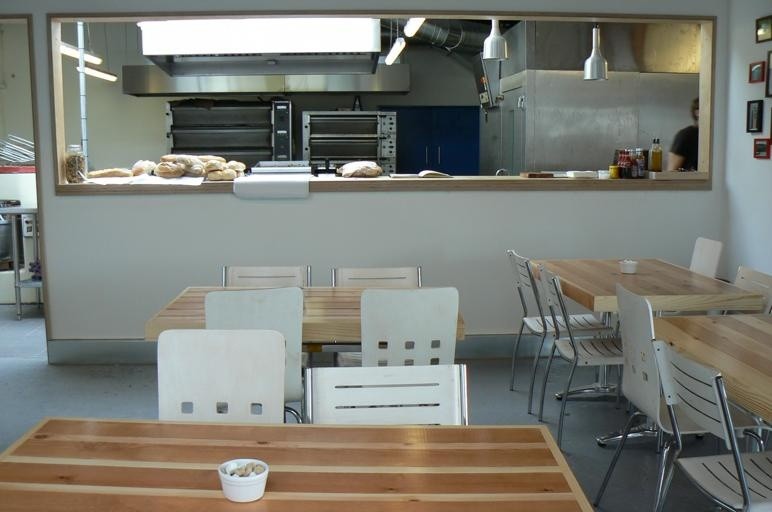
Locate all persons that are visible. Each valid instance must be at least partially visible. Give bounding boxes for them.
[662,95,700,172]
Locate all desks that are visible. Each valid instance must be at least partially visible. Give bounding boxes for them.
[141,286,466,350]
[1,413,599,511]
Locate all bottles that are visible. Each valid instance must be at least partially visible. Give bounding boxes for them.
[649,138,662,172]
[617,147,646,178]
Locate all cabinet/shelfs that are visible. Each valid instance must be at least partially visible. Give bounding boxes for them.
[0,206,47,323]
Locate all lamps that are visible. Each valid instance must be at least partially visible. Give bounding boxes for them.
[579,23,608,82]
[402,16,427,39]
[383,19,407,69]
[479,19,510,64]
[76,66,118,83]
[60,22,106,68]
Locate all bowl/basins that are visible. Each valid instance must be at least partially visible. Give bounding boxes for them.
[618,261,638,274]
[218,458,268,502]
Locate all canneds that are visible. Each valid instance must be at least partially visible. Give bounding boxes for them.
[65,145,85,184]
[609,165,620,179]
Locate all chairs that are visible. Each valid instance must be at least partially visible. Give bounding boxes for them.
[302,362,470,429]
[152,326,289,424]
[202,286,308,424]
[220,262,317,367]
[329,264,424,365]
[335,283,460,408]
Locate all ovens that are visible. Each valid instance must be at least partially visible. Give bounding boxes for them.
[301,111,397,174]
[165,98,294,167]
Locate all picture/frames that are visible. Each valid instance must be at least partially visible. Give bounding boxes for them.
[745,13,772,162]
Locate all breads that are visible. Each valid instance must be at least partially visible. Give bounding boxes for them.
[85,154,247,180]
[337,160,383,177]
